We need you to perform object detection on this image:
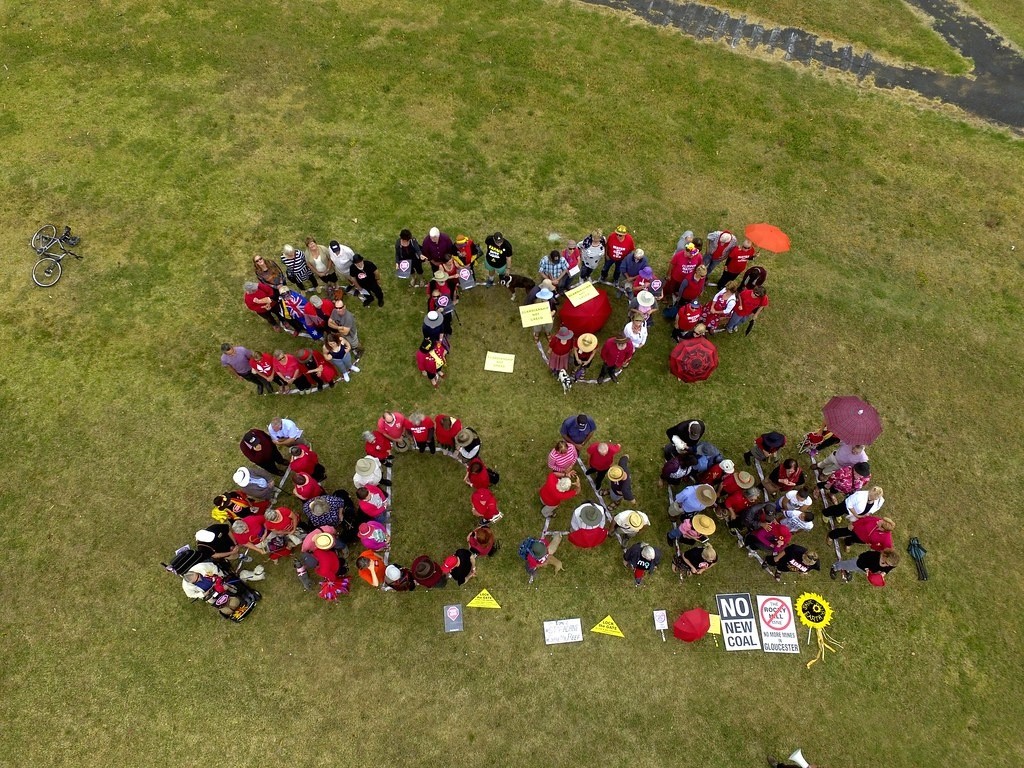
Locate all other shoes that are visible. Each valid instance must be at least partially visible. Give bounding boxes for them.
[767,755,778,768]
[811,462,853,581]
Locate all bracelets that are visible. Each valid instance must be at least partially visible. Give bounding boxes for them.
[507,268,510,269]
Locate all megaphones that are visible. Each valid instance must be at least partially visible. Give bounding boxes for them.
[789,747,810,768]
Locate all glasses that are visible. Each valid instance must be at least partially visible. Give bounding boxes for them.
[693,330,702,335]
[807,554,817,561]
[743,246,751,249]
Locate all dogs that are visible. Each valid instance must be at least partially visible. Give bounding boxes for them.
[293,559,313,593]
[539,531,566,577]
[556,368,573,395]
[240,564,266,583]
[499,273,535,301]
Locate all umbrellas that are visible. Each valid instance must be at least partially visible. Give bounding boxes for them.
[672,607,710,643]
[669,337,719,383]
[745,223,791,253]
[567,526,609,549]
[558,289,612,338]
[821,396,883,446]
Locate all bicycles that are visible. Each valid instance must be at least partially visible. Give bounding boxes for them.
[31,224,82,287]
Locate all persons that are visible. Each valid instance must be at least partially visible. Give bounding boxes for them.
[658,420,900,582]
[393,227,513,389]
[524,414,661,584]
[352,412,499,593]
[523,225,664,384]
[182,417,349,603]
[662,229,769,345]
[221,237,384,396]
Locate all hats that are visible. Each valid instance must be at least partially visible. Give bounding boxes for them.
[626,512,644,529]
[734,470,756,489]
[855,462,870,476]
[693,514,716,536]
[719,459,736,474]
[696,484,717,508]
[766,503,777,518]
[721,233,731,242]
[764,432,784,448]
[855,462,869,477]
[692,299,698,307]
[689,423,703,440]
[194,224,662,581]
[696,443,719,457]
